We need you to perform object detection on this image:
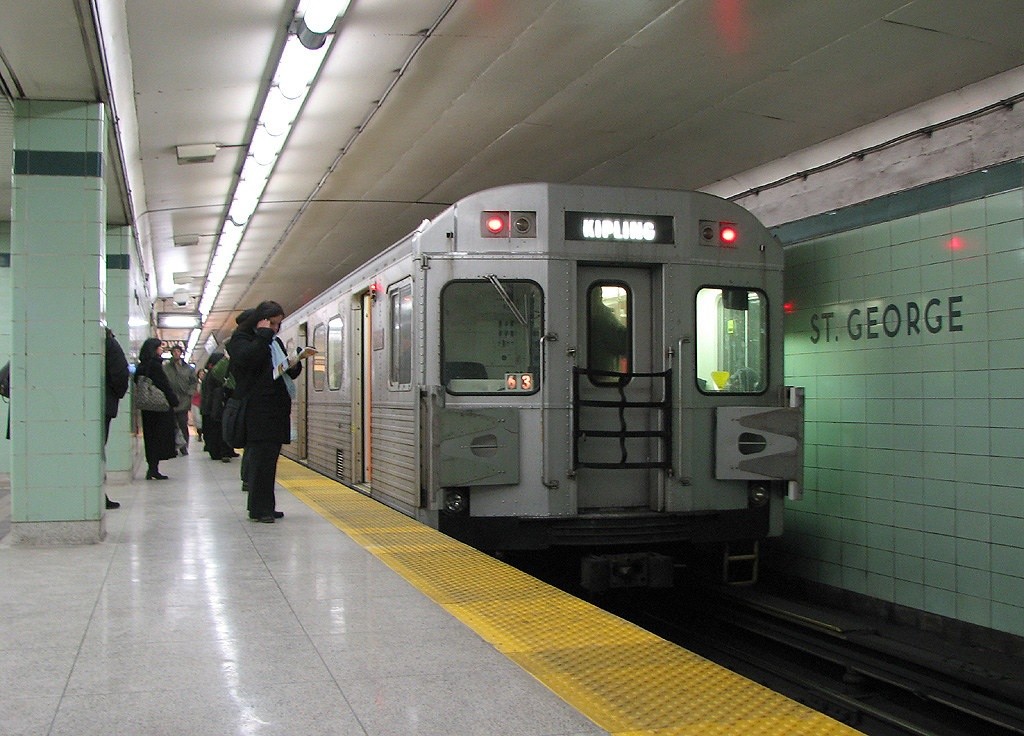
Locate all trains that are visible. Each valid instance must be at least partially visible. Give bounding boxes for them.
[270,182,805,586]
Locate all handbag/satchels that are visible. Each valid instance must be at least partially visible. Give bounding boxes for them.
[222,398,247,449]
[211,356,236,390]
[134,376,169,412]
[0,361,10,398]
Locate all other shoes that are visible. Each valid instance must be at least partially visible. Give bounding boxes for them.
[222,456,231,462]
[198,437,202,442]
[105,494,120,509]
[242,482,249,491]
[273,511,284,518]
[180,446,188,455]
[232,452,240,457]
[253,515,274,522]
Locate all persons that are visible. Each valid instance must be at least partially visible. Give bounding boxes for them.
[200,352,240,460]
[104,329,129,509]
[162,344,197,455]
[236,308,256,491]
[591,287,626,381]
[192,369,207,442]
[230,300,301,522]
[135,338,179,480]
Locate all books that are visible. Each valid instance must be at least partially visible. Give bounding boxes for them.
[272,346,318,380]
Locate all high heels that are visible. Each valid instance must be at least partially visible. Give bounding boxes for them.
[146,469,168,480]
